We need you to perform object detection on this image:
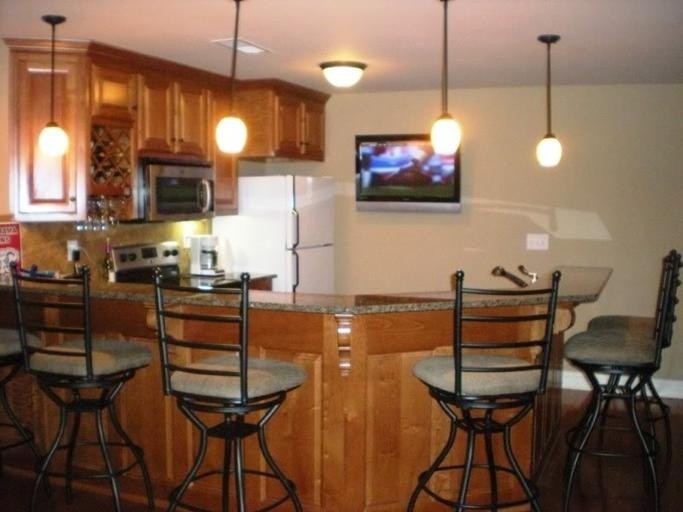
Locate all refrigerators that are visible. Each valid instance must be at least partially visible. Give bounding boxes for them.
[238,174,335,294]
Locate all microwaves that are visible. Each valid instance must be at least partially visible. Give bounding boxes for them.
[144,163,214,222]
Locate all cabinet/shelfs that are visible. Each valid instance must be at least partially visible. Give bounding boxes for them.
[237,86,331,166]
[136,72,215,164]
[212,87,243,213]
[89,64,140,176]
[5,50,86,224]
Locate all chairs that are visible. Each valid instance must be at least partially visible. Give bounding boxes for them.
[0,326,54,505]
[6,260,156,512]
[403,270,562,510]
[143,269,307,512]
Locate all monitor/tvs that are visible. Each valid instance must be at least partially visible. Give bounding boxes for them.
[353,133,462,215]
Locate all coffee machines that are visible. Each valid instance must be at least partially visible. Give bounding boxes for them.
[190,236,225,277]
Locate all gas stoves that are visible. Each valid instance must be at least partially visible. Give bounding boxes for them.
[108,240,181,287]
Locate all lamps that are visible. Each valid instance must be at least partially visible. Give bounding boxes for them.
[428,0,465,155]
[534,33,565,167]
[29,16,72,163]
[318,59,369,89]
[218,3,250,155]
